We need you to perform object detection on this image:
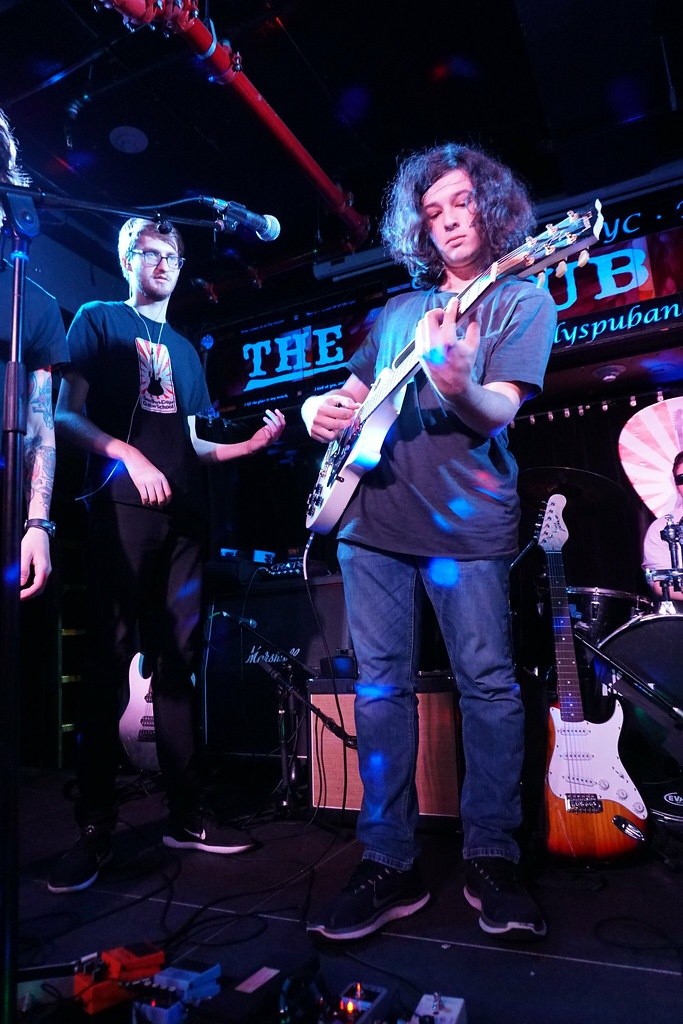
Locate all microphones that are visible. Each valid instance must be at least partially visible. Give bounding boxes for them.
[199,195,280,242]
[221,612,257,629]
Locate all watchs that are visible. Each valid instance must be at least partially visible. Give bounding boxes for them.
[24,519,56,540]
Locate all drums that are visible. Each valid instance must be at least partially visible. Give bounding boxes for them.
[585,612,683,825]
[563,584,654,651]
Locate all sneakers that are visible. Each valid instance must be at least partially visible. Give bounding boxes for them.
[314,857,431,943]
[463,864,549,938]
[162,814,254,853]
[49,825,115,894]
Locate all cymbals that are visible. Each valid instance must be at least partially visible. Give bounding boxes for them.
[517,466,630,512]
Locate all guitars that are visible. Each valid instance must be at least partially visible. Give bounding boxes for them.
[117,653,160,769]
[519,492,654,868]
[305,196,604,536]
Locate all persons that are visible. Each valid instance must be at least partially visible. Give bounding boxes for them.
[301,143,557,939]
[47,217,286,892]
[641,451,683,601]
[0,108,72,602]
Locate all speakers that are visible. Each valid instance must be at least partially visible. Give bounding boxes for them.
[306,676,466,828]
[203,575,355,768]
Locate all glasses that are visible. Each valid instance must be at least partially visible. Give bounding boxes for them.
[129,248,186,271]
[674,473,683,487]
